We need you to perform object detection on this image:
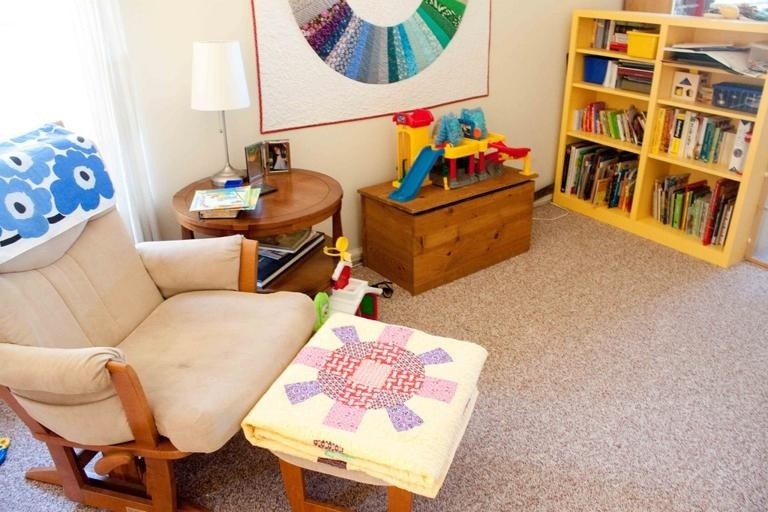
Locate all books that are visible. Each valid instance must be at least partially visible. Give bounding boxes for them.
[256,227,325,289]
[187,186,260,220]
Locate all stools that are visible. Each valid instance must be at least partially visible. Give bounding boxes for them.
[278,456,414,512]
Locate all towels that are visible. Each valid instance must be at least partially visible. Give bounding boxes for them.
[1,123,117,265]
[240,310,489,499]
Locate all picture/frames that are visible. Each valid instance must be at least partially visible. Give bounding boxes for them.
[244,139,290,183]
[249,0,490,132]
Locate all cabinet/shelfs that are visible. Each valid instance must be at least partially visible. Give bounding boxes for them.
[553,9,768,267]
[357,165,535,296]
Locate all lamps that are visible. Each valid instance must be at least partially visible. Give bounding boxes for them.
[191,41,250,187]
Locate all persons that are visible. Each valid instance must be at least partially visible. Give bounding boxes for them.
[270,143,288,170]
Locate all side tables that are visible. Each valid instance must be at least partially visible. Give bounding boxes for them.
[172,168,343,296]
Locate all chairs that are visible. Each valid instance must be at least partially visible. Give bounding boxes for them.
[0,121,316,512]
